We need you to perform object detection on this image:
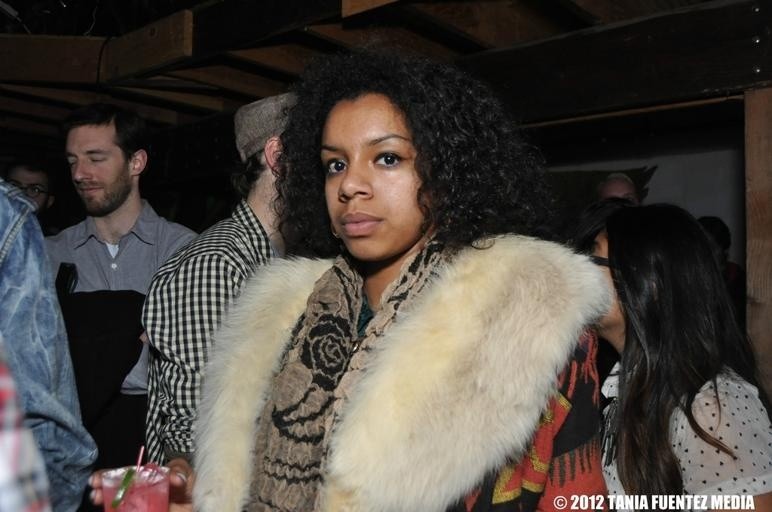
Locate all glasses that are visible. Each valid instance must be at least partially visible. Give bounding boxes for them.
[589,255,610,267]
[5,178,49,199]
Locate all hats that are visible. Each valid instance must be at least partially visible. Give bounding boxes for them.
[234,90,300,163]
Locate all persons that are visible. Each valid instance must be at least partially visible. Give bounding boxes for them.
[88,28,613,511]
[139,88,302,471]
[43,104,201,512]
[5,153,63,240]
[695,213,746,337]
[593,170,637,207]
[585,203,772,511]
[0,177,99,512]
